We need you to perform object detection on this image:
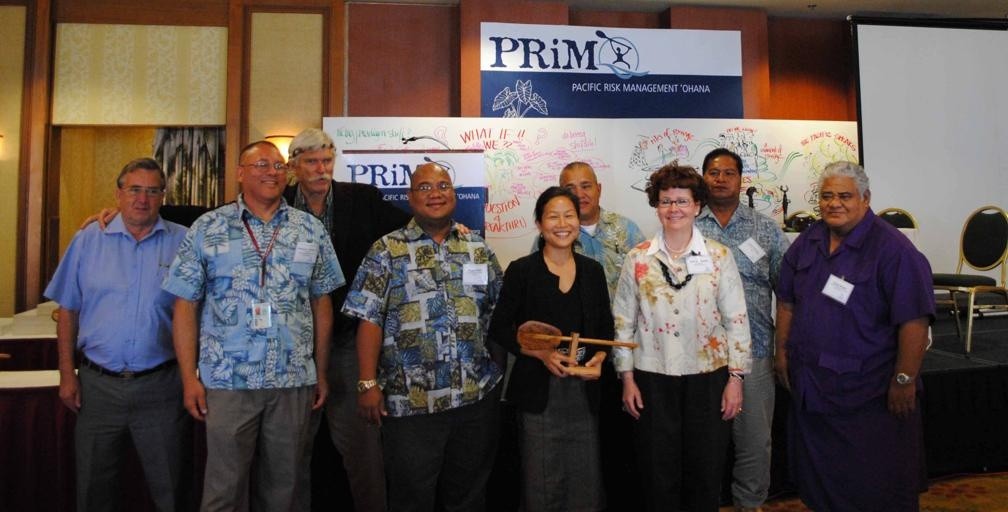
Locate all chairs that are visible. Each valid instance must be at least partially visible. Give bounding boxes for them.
[877,207,918,250]
[933,205,1008,358]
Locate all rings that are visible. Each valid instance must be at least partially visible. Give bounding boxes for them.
[739,407,744,412]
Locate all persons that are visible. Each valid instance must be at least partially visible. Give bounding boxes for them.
[532,162,647,512]
[44,156,193,512]
[165,139,347,512]
[80,126,471,511]
[772,163,937,512]
[340,164,507,512]
[692,147,787,512]
[483,187,615,512]
[610,163,752,512]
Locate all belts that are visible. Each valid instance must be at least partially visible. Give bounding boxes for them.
[82,357,177,381]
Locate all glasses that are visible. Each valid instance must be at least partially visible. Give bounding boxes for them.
[413,181,450,192]
[241,159,286,171]
[120,187,163,195]
[659,198,691,208]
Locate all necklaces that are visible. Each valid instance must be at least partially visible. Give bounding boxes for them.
[658,249,701,291]
[657,227,701,290]
[661,232,693,259]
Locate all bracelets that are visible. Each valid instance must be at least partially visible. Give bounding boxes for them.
[727,378,745,384]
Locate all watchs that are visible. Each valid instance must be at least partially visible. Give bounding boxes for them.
[891,373,918,385]
[357,381,379,391]
